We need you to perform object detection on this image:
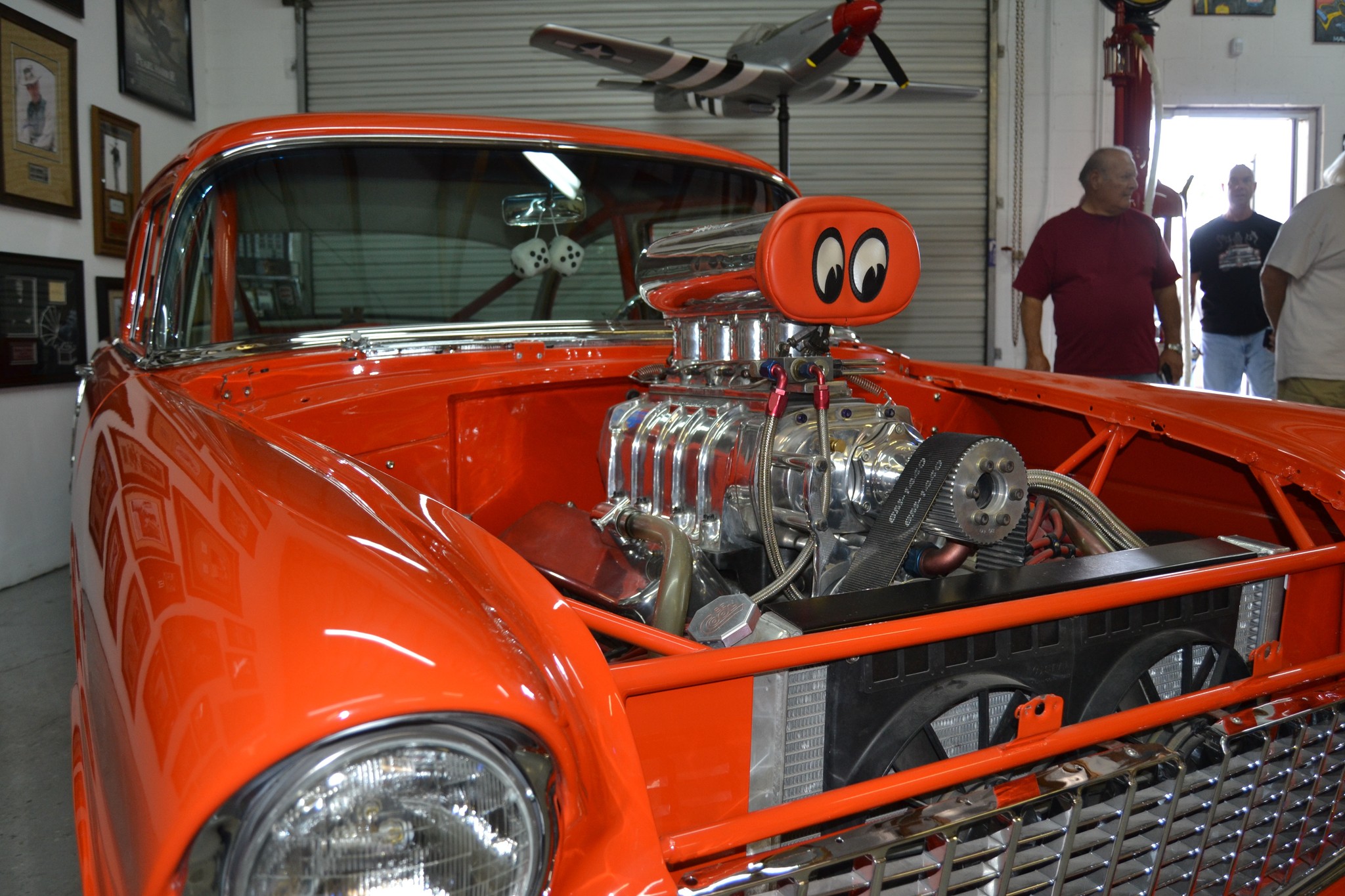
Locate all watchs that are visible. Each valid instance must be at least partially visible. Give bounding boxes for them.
[1166,343,1183,355]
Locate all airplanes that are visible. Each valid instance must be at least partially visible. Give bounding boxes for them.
[531,0,979,120]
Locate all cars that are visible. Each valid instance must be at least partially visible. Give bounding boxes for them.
[71,112,1345,896]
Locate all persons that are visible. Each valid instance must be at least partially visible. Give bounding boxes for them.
[16,64,57,153]
[1011,146,1185,386]
[111,142,122,193]
[1257,150,1345,411]
[1189,164,1285,401]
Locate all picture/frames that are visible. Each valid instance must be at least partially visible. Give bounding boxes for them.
[90,104,143,262]
[41,0,86,19]
[115,0,197,122]
[94,276,126,346]
[0,250,89,390]
[0,0,82,220]
